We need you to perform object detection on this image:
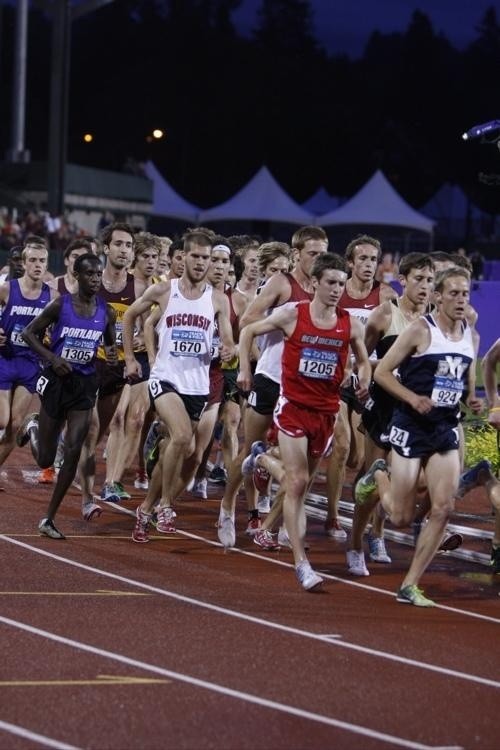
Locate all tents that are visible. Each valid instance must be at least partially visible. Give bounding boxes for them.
[68,160,435,249]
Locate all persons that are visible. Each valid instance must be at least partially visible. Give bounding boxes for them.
[0,192,499,608]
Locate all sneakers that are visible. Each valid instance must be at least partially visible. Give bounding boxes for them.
[39,520,65,539]
[396,585,435,608]
[346,549,369,577]
[55,433,65,464]
[295,561,323,591]
[458,459,491,500]
[355,458,388,503]
[102,420,311,552]
[439,529,463,552]
[38,467,56,483]
[412,522,426,545]
[366,527,392,563]
[489,547,500,573]
[324,518,347,538]
[82,502,102,521]
[16,413,40,447]
